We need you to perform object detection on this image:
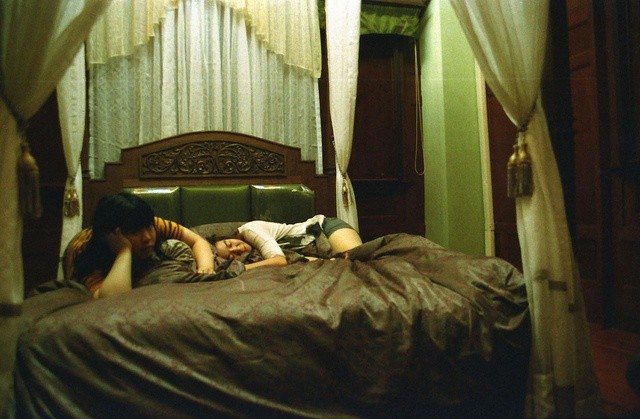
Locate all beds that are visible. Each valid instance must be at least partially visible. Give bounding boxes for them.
[10,128,535,418]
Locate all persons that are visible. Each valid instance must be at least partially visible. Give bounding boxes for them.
[62,190,216,300]
[206,214,363,274]
